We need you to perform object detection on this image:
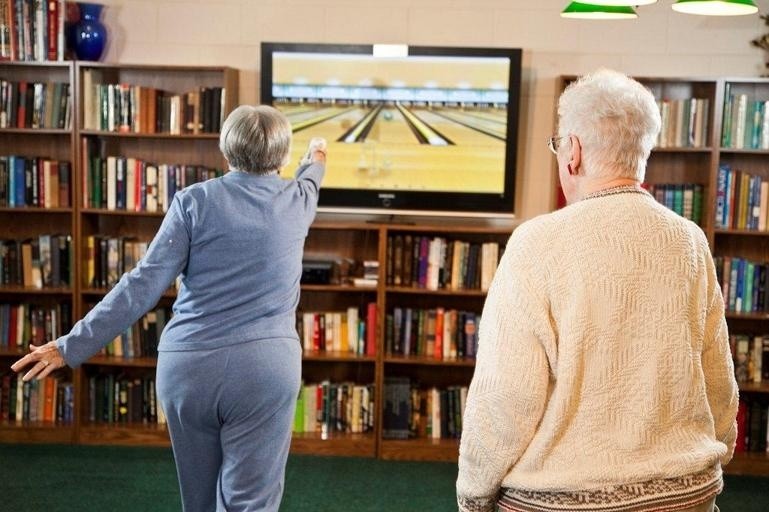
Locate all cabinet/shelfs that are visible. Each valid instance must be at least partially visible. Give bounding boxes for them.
[0,61,79,446]
[698,75,769,479]
[75,61,240,447]
[555,75,718,259]
[376,218,524,462]
[286,221,386,457]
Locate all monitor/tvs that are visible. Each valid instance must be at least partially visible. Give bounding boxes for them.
[261,42,522,226]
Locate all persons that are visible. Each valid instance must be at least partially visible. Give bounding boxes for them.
[453,68,741,511]
[10,101,329,511]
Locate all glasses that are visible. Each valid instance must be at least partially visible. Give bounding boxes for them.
[547,136,564,154]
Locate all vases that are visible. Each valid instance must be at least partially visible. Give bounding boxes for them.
[70,2,107,60]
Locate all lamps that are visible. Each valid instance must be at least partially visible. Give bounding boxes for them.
[674,2,756,18]
[560,1,640,19]
